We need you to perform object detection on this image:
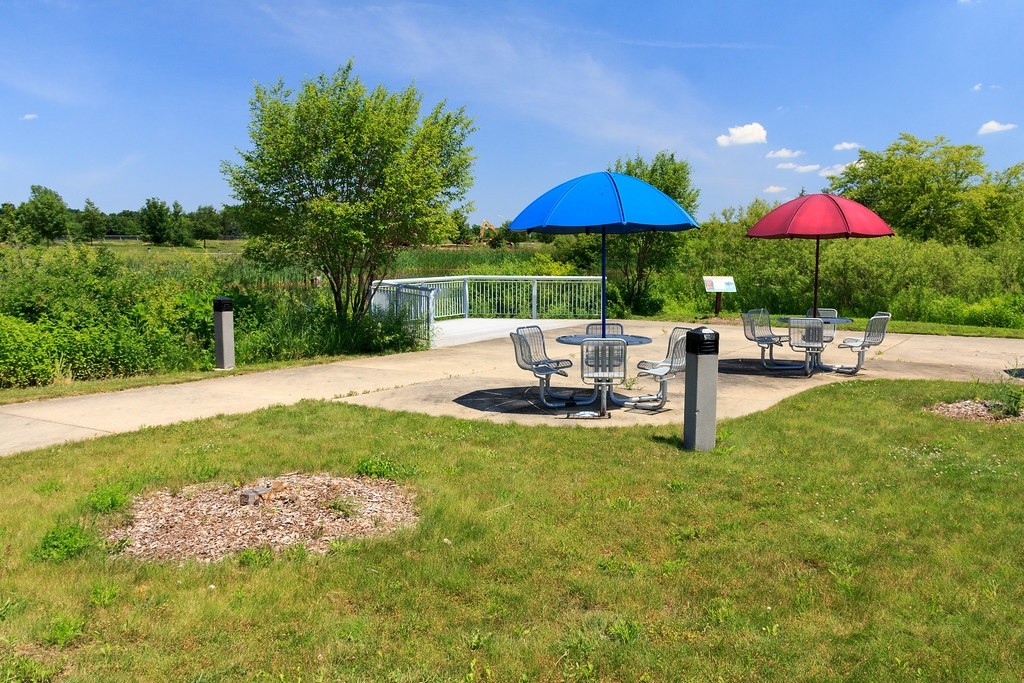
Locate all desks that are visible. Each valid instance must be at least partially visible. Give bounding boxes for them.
[556,334,652,415]
[777,317,854,375]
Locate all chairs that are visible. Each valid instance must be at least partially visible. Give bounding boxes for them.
[587,322,625,367]
[802,308,838,367]
[511,333,568,407]
[516,325,573,399]
[637,326,692,400]
[789,318,826,374]
[581,338,627,415]
[637,335,686,410]
[740,308,790,369]
[838,311,891,374]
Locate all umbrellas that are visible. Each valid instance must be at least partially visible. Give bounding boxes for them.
[508,172,700,338]
[744,194,896,318]
